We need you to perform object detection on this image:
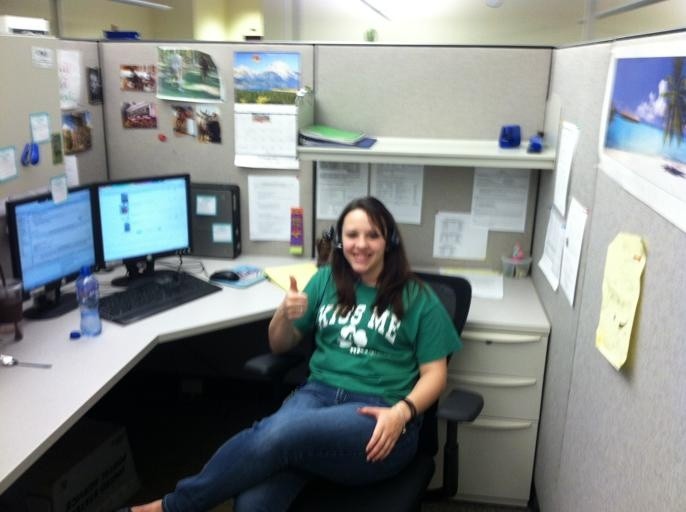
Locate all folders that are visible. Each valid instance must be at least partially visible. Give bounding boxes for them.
[298,123,376,149]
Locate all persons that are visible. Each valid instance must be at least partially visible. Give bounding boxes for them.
[87,72,102,101]
[122,194,467,512]
[121,101,133,124]
[198,55,210,81]
[172,105,223,146]
[167,53,189,92]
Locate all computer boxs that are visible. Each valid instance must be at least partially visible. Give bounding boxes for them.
[192,182,242,259]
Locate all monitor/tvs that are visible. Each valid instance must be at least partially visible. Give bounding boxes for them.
[6,184,101,320]
[94,174,193,286]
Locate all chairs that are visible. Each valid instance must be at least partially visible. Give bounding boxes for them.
[242,271,486,512]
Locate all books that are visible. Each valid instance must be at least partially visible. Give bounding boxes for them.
[297,135,377,151]
[302,124,368,145]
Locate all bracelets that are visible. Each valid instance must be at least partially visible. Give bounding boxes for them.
[401,397,419,422]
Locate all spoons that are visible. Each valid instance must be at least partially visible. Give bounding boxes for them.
[1,355,52,369]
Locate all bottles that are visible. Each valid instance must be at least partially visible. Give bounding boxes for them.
[76,265,103,336]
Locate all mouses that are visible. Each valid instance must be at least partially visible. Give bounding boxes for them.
[211,271,239,281]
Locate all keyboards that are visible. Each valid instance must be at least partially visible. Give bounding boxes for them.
[99,271,222,327]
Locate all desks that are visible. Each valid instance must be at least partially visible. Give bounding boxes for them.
[0,256,553,512]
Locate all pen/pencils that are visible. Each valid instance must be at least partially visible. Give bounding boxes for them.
[321,226,335,241]
